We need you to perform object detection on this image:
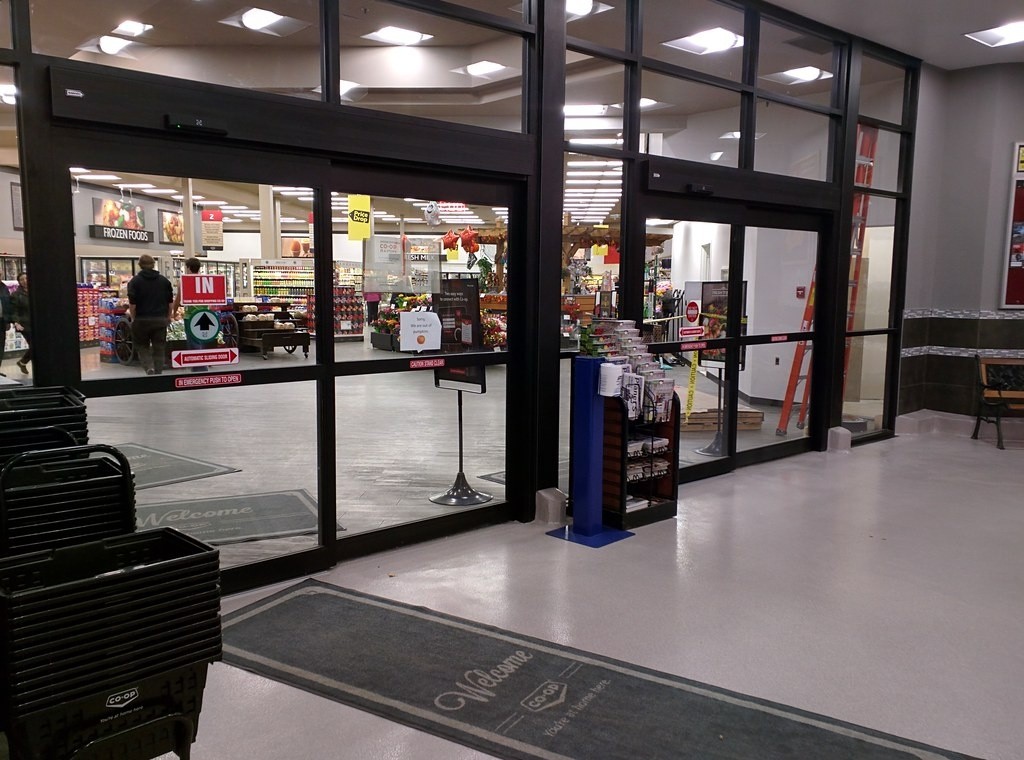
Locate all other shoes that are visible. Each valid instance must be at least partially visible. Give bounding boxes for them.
[17,359,30,374]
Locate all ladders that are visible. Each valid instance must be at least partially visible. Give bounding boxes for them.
[774,121,882,437]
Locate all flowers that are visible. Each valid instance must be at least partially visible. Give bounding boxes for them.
[370,294,432,334]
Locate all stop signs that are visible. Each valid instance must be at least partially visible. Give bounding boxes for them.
[686,301,699,323]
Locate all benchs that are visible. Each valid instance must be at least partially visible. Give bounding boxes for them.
[971,352,1024,450]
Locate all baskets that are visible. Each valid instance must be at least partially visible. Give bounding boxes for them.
[0,382,222,759]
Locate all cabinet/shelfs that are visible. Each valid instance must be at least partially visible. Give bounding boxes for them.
[233,302,310,361]
[580,325,681,531]
[97,309,127,363]
[239,258,363,327]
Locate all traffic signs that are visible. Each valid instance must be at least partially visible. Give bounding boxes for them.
[348,210,370,224]
[171,347,239,369]
[679,325,705,338]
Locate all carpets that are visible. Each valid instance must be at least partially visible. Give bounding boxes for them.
[135,488,347,546]
[216,575,988,760]
[475,458,695,495]
[88,443,242,489]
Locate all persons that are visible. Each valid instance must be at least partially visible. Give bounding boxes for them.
[613,276,619,294]
[85,274,93,284]
[127,254,174,374]
[0,265,12,377]
[363,280,382,327]
[390,282,417,309]
[171,257,210,371]
[9,272,33,374]
